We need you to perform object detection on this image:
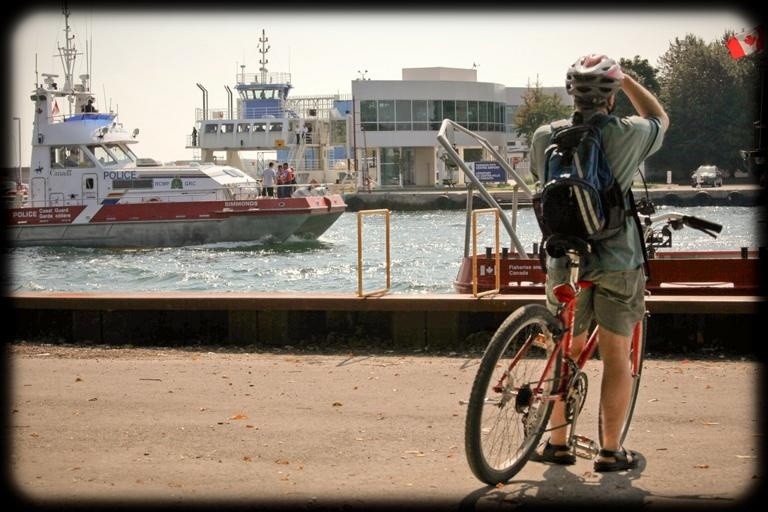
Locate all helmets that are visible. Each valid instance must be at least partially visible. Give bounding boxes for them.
[566,54,623,100]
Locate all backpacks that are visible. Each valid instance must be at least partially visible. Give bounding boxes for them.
[530,114,627,244]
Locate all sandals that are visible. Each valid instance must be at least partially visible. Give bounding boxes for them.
[531,433,577,464]
[593,446,638,472]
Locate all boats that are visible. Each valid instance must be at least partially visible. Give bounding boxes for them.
[5,8,349,248]
[457,243,758,296]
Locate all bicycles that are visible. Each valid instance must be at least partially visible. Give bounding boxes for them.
[466,208,723,487]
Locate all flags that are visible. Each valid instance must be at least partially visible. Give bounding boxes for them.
[723,23,763,58]
[50,99,60,122]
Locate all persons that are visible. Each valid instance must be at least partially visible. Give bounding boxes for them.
[259,161,276,197]
[80,96,97,112]
[294,122,301,144]
[300,124,308,145]
[522,51,673,475]
[290,166,297,192]
[65,147,80,168]
[277,162,292,197]
[275,165,283,197]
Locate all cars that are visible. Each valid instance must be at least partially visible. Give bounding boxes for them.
[690,164,723,186]
